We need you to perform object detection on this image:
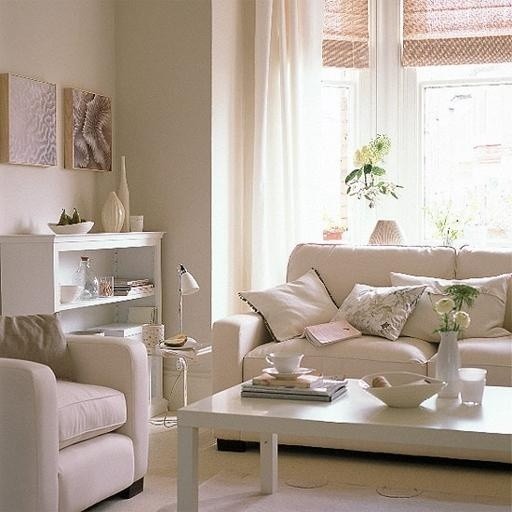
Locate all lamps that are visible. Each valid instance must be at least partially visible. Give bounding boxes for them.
[160,263,200,350]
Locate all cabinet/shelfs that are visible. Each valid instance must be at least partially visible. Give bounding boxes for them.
[0,231,168,419]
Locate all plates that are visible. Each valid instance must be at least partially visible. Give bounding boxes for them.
[262,367,315,379]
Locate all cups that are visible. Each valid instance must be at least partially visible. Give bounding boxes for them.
[130,215,144,232]
[265,351,305,372]
[457,366,487,404]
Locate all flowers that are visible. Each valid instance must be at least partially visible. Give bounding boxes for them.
[344,132,405,208]
[433,285,481,331]
[323,207,349,232]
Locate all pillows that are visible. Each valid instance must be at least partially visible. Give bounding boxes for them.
[236,269,512,342]
[0,312,77,382]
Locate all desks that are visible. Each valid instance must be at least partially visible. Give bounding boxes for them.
[176,373,512,512]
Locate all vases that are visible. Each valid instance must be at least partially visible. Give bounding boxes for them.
[322,229,345,240]
[367,220,408,246]
[118,155,130,231]
[100,190,126,233]
[436,331,460,399]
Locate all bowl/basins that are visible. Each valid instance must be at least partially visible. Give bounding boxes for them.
[358,371,449,407]
[48,221,95,236]
[60,284,85,305]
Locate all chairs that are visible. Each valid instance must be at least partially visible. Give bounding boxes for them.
[1,333,151,512]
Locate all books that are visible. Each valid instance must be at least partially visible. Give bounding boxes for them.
[241,373,348,404]
[297,319,361,348]
[105,274,155,297]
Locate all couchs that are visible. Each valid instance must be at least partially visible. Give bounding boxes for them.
[211,243,512,451]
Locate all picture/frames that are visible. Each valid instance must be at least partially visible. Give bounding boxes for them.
[1,73,59,168]
[64,87,113,174]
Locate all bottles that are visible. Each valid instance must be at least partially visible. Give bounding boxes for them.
[72,257,99,300]
